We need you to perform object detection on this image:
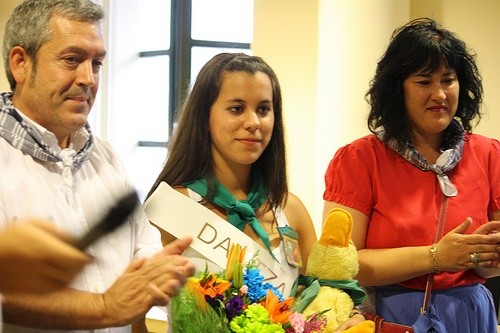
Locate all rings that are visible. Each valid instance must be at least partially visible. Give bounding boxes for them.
[471,253,477,263]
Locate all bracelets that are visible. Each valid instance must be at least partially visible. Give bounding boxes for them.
[430,244,440,273]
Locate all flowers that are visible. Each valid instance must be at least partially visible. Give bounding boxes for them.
[169,242,354,333]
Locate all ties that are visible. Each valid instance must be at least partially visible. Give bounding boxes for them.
[0,90,95,188]
[182,169,283,264]
[375,115,466,197]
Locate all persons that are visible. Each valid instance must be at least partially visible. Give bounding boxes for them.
[0,0,196,333]
[323,17,500,333]
[144,52,366,333]
